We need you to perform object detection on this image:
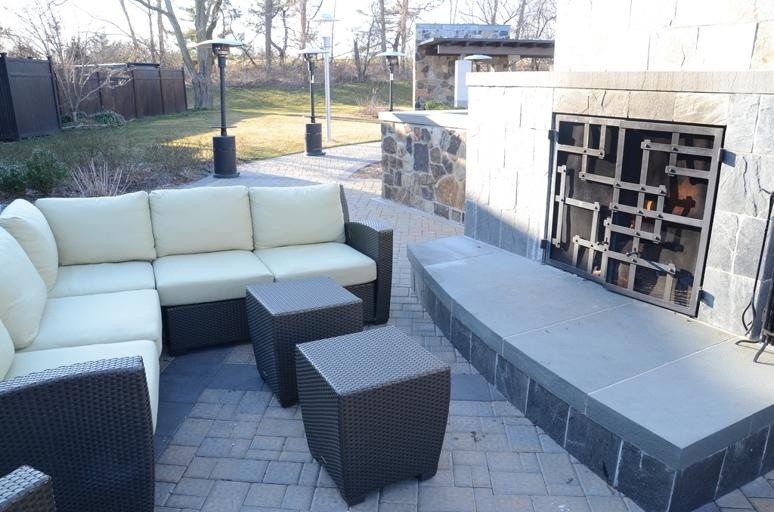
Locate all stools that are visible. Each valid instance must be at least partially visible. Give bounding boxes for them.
[245,277,363,409]
[295,327,451,506]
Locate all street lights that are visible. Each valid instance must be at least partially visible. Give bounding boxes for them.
[295,48,326,158]
[194,38,250,180]
[380,50,404,111]
[318,18,335,145]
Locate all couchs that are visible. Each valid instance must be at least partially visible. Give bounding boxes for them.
[0,190,162,512]
[149,184,394,358]
